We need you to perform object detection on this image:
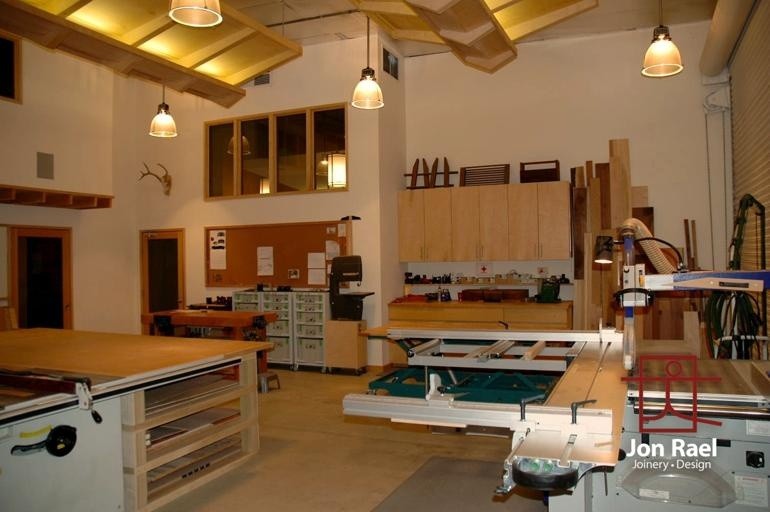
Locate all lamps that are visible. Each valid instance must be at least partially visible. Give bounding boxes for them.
[315,133,328,176]
[594,234,688,273]
[259,176,270,194]
[350,15,384,111]
[327,132,346,187]
[148,78,177,138]
[169,0,223,27]
[226,134,251,155]
[641,0,684,78]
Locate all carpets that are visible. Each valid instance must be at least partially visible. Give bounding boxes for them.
[371,455,550,512]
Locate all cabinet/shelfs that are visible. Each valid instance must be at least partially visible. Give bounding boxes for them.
[386,303,573,368]
[231,288,368,376]
[397,180,573,261]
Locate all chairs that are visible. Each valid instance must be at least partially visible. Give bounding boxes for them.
[153,315,190,337]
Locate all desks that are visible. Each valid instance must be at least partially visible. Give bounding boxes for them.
[193,303,226,338]
[141,310,277,386]
[0,328,273,512]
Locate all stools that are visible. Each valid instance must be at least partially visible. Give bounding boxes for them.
[256,371,280,393]
